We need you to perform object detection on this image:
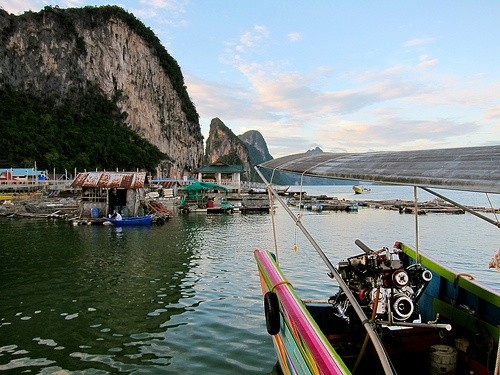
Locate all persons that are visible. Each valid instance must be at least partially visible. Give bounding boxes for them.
[109,210,122,220]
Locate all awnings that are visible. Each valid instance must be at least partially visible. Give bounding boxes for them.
[257,145,500,197]
[182,181,230,192]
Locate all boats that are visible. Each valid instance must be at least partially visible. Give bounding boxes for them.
[253,145,500,375]
[101,215,155,226]
[353,186,372,195]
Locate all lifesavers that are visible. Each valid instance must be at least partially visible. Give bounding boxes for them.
[264,292,281,335]
